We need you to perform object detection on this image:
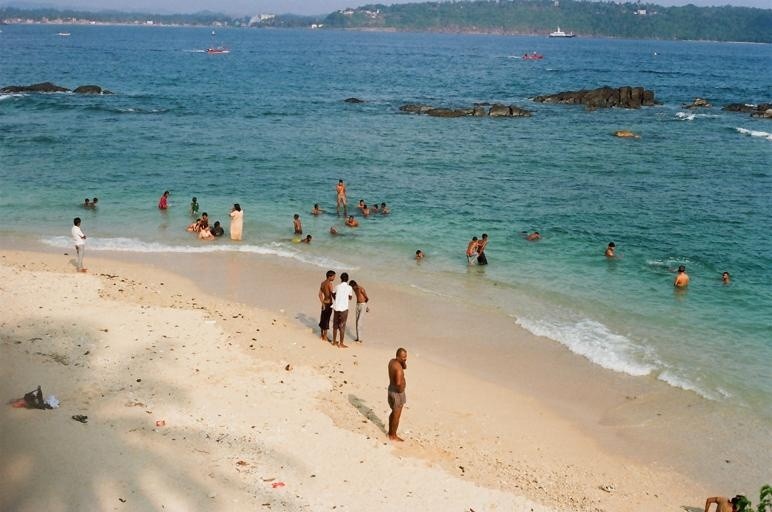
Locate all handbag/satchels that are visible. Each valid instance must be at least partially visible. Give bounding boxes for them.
[23,385,46,410]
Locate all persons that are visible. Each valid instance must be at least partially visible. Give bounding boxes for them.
[186,196,244,243]
[71,197,99,273]
[158,189,170,210]
[386,349,412,442]
[673,266,690,287]
[722,272,730,285]
[287,179,389,242]
[476,232,491,264]
[414,249,425,258]
[705,494,747,512]
[318,270,370,351]
[467,236,481,266]
[605,242,618,258]
[526,231,542,241]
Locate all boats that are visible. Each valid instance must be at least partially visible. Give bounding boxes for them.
[206,47,230,53]
[522,52,545,59]
[57,32,72,35]
[211,29,217,35]
[548,27,575,37]
